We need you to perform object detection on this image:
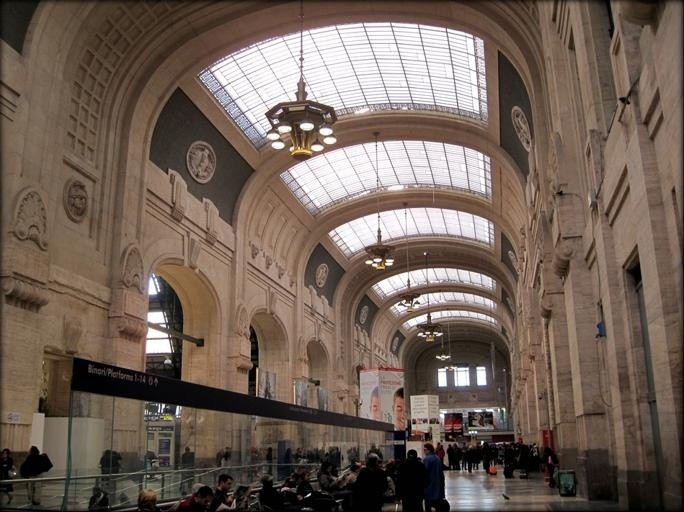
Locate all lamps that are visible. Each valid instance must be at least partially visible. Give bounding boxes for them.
[397,203,420,312]
[417,248,442,343]
[444,316,457,372]
[264,0,338,161]
[365,132,395,270]
[432,310,452,362]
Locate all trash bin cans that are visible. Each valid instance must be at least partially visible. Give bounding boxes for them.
[559,470,576,496]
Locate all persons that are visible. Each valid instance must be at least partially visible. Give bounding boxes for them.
[370,386,381,420]
[27,445,41,505]
[88,441,445,512]
[469,412,493,427]
[436,442,558,480]
[391,387,405,431]
[0,449,13,505]
